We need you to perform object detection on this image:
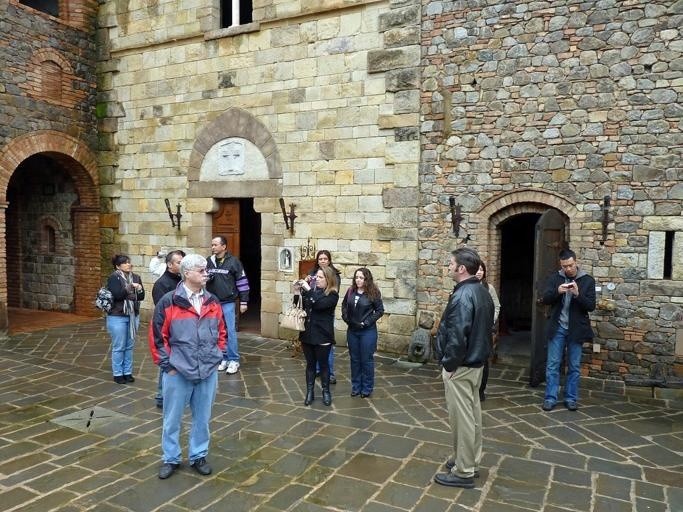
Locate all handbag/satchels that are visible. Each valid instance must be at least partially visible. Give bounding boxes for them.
[95,287,114,315]
[280,304,308,331]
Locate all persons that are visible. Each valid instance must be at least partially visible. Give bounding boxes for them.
[476,259,502,402]
[149,250,168,300]
[204,236,250,376]
[291,264,339,407]
[341,264,385,398]
[102,253,146,384]
[148,254,228,480]
[300,248,342,385]
[542,248,597,411]
[434,247,495,490]
[152,250,185,409]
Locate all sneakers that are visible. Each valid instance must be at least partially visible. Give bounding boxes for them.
[114,375,133,384]
[543,401,555,411]
[330,375,336,384]
[191,457,211,474]
[159,463,179,479]
[219,360,240,373]
[565,400,577,410]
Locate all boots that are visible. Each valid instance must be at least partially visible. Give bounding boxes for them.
[322,382,331,405]
[306,382,314,405]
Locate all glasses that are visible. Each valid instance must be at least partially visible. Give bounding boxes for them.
[189,268,207,274]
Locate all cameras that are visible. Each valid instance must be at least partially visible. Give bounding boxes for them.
[563,283,573,288]
[294,280,304,287]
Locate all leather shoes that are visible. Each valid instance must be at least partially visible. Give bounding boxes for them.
[434,460,478,488]
[351,391,368,397]
